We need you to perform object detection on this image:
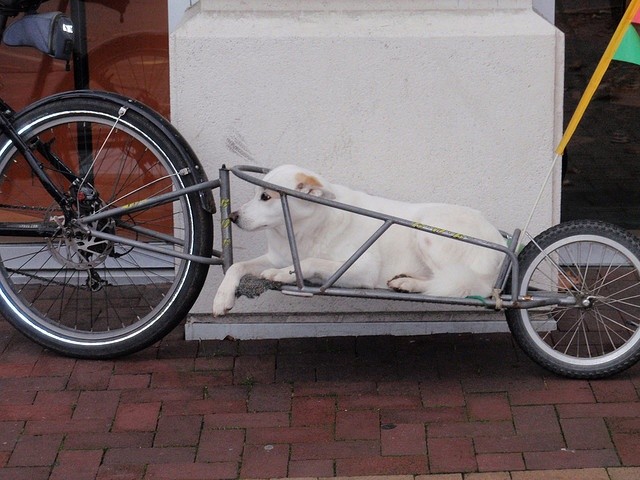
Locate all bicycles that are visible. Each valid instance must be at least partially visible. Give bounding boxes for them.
[0,0,640,379]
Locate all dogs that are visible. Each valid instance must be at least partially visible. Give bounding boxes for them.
[212,164,508,318]
